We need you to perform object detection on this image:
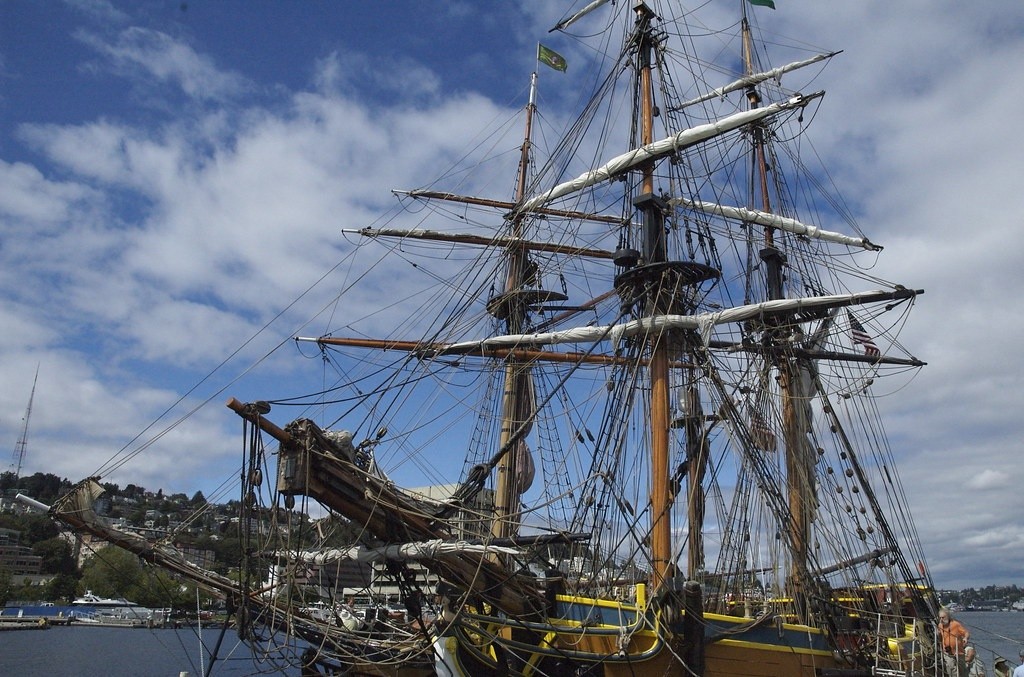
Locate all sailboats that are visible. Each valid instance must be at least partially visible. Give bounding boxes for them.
[10,0,1024,677]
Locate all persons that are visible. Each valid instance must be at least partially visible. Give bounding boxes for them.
[938,608,970,677]
[1013,650,1024,677]
[992,656,1014,677]
[965,642,984,677]
[340,599,358,630]
[741,572,764,600]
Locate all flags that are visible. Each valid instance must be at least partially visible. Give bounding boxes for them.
[748,0,776,10]
[539,44,567,74]
